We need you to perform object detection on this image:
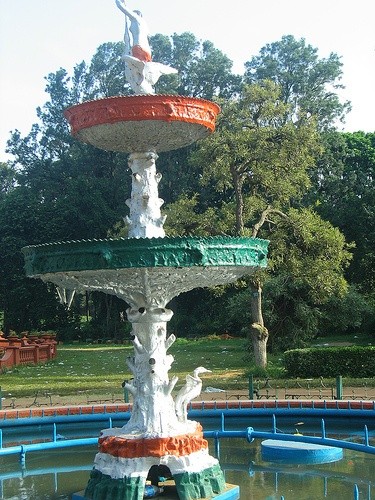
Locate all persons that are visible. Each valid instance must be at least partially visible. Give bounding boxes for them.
[115,0,153,95]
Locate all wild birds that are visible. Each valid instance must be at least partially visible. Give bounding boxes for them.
[294,421,304,432]
[175,366,212,424]
